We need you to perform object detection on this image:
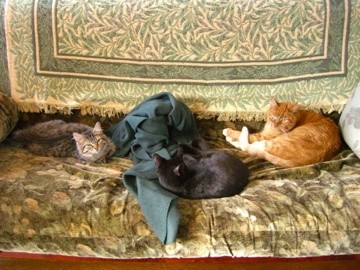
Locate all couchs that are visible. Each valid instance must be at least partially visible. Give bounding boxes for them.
[0,0,360,262]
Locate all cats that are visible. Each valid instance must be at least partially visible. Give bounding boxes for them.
[222,98,343,169]
[4,118,117,166]
[152,137,248,201]
